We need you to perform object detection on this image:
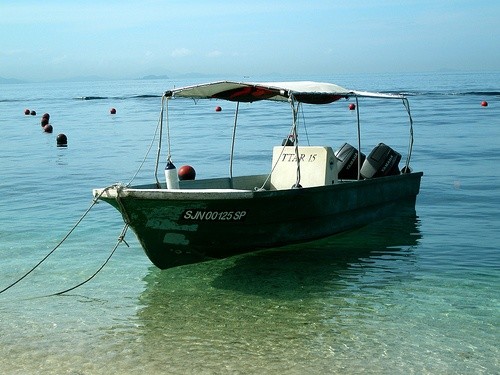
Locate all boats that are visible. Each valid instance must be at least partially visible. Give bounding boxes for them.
[91,77,421,271]
